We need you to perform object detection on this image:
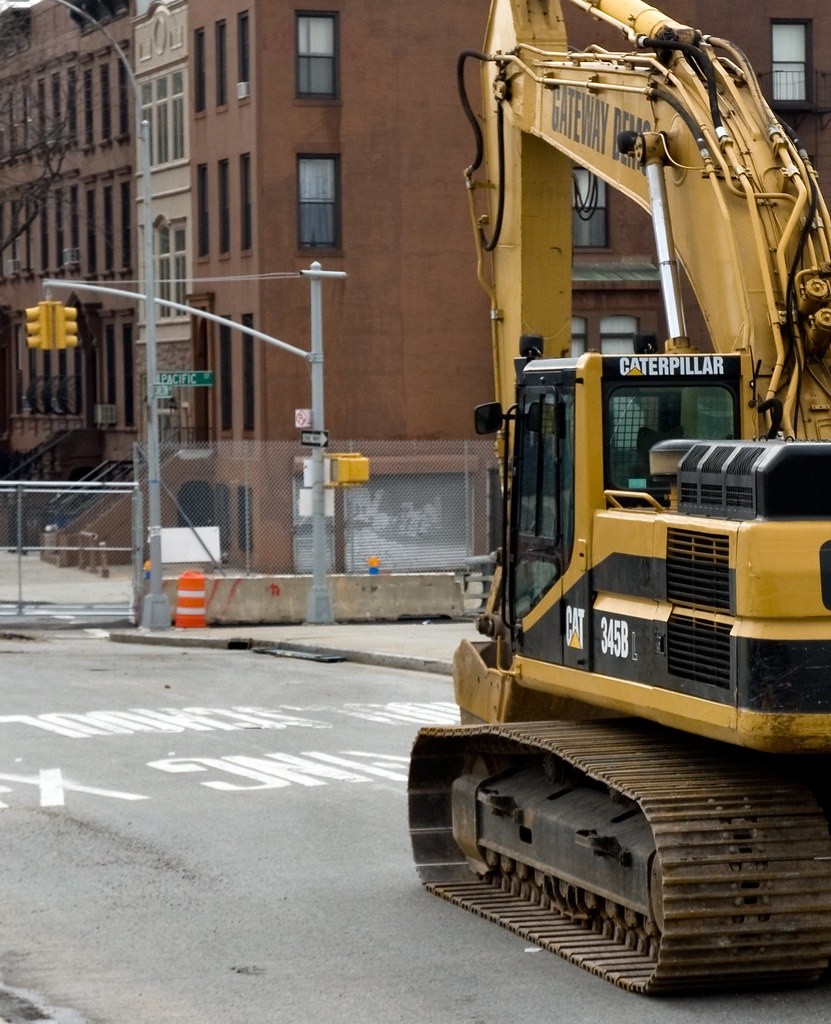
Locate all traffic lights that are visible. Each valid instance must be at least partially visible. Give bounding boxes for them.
[50,302,78,350]
[25,301,52,351]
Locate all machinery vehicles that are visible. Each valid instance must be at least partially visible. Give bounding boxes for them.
[410,0,831,999]
